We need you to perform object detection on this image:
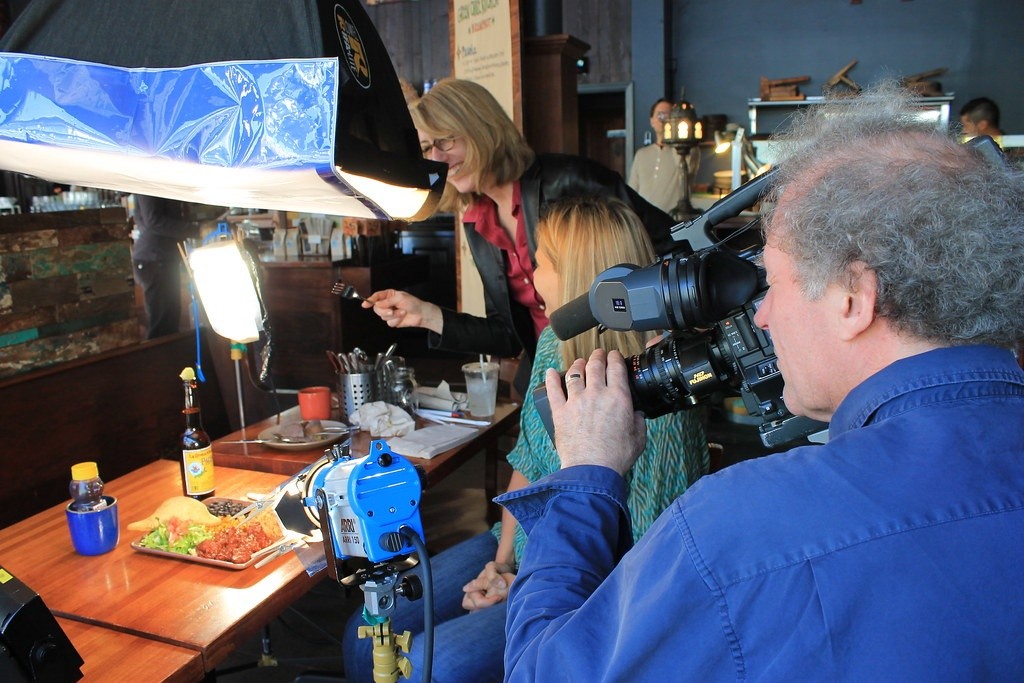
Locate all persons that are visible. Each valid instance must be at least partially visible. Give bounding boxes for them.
[956,96,1024,169]
[487,71,1023,683]
[336,194,716,683]
[361,75,690,398]
[625,97,701,223]
[127,193,225,343]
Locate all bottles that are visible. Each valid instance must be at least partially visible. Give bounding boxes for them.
[69,461,107,512]
[179,367,215,501]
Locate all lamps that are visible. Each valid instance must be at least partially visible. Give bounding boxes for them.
[656,84,705,223]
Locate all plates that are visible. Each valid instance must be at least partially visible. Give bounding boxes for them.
[258,420,348,450]
[130,497,281,569]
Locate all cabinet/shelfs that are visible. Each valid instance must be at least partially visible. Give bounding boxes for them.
[742,90,954,137]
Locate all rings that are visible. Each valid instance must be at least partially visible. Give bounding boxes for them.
[563,372,585,387]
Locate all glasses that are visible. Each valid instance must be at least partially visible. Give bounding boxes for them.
[421,135,455,156]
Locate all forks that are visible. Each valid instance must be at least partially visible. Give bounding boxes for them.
[330,283,376,307]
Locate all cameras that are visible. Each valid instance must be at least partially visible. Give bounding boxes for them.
[272,440,424,590]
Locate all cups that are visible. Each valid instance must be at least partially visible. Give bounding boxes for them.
[299,386,331,420]
[461,362,500,423]
[386,367,419,422]
[66,495,119,556]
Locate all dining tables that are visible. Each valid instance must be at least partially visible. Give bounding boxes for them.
[52,614,208,683]
[218,388,520,525]
[0,455,328,670]
